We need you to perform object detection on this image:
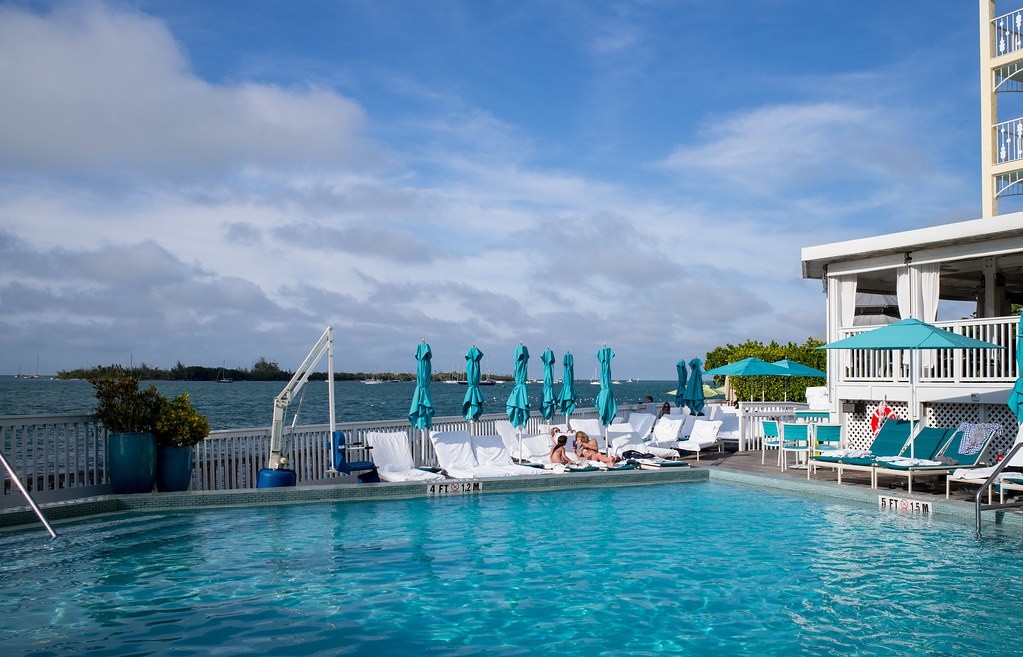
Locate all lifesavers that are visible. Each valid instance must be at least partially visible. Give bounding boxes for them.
[872,408,896,436]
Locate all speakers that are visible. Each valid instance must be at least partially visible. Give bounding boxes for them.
[843,403,864,413]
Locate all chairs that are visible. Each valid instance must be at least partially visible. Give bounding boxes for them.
[367,430,446,481]
[333,430,380,473]
[429,404,760,478]
[782,424,811,471]
[808,418,1023,504]
[760,421,791,467]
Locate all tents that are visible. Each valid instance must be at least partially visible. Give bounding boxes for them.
[666,382,719,397]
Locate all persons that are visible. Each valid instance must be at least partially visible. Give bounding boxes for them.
[573,431,587,453]
[550,426,560,447]
[658,401,670,419]
[576,434,620,468]
[550,435,576,465]
[646,395,653,403]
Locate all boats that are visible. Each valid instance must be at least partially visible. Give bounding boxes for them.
[524,376,565,385]
[611,380,622,385]
[626,378,634,383]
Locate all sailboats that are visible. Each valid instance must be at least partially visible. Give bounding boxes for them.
[215,360,234,383]
[14,354,55,381]
[589,364,601,386]
[441,359,507,386]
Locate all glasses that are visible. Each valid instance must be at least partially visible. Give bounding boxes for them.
[553,429,560,433]
[664,405,668,408]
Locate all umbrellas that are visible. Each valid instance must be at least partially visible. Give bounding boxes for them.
[408,338,435,465]
[769,356,826,402]
[1007,308,1023,424]
[461,341,485,435]
[506,339,530,464]
[538,345,558,425]
[699,357,809,441]
[816,314,1007,459]
[683,355,705,413]
[673,356,687,414]
[595,341,618,457]
[557,348,577,430]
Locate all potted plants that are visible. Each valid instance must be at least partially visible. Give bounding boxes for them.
[88,366,213,494]
